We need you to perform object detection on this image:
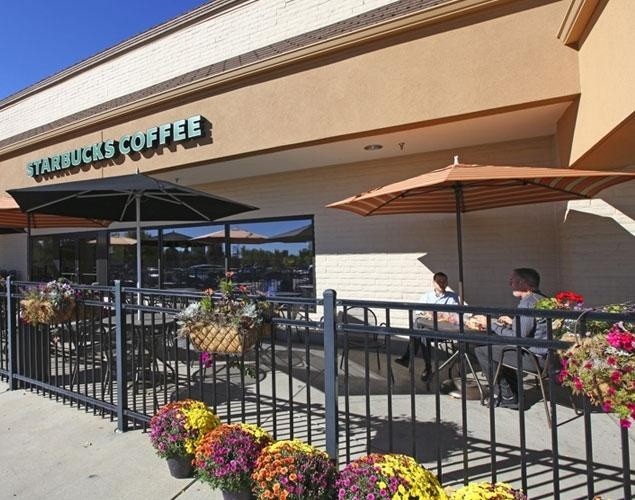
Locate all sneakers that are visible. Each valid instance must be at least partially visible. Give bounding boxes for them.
[497,393,519,409]
[394,355,410,368]
[421,369,432,381]
[488,393,501,408]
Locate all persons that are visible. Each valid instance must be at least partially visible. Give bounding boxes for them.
[474,268,553,408]
[395,272,459,380]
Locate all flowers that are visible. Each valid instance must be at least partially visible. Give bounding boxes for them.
[169,271,271,334]
[149,398,222,460]
[556,322,635,429]
[191,422,276,490]
[254,440,338,499]
[335,452,446,499]
[553,290,584,306]
[449,481,528,500]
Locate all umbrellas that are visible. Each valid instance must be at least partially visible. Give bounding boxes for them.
[0,197,112,279]
[326,154,635,377]
[4,170,259,320]
[86,236,137,246]
[268,223,314,242]
[187,228,267,244]
[142,229,199,247]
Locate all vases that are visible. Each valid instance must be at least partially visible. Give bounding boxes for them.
[162,451,196,478]
[220,487,257,500]
[187,319,263,354]
[580,377,610,408]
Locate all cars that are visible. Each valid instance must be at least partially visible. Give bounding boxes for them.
[118,261,311,297]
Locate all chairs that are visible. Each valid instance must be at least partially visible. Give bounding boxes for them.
[0,264,198,395]
[417,305,600,432]
[338,306,396,381]
